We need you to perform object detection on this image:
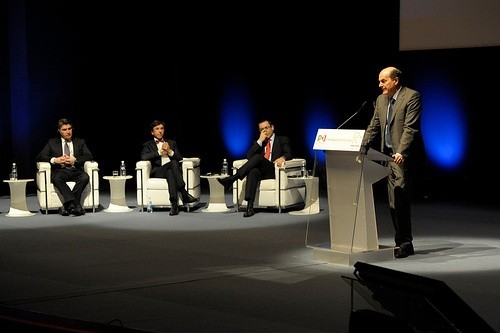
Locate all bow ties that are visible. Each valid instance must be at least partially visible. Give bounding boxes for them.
[155,138,164,144]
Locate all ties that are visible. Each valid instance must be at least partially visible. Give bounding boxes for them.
[386,97,395,145]
[263,138,270,159]
[65,139,70,158]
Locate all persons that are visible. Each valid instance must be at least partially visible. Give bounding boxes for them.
[356,66,422,257]
[139,120,199,216]
[216,118,291,218]
[39,117,93,214]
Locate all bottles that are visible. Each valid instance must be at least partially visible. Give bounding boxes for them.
[222,158,228,176]
[300,160,307,177]
[119,161,126,177]
[11,162,18,180]
[146,197,153,212]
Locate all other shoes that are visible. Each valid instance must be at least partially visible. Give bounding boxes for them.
[182,193,200,203]
[169,204,179,215]
[243,205,255,216]
[216,178,232,191]
[61,204,85,216]
[394,241,414,258]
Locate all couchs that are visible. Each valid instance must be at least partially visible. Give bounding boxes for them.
[35,161,99,213]
[233,158,308,215]
[134,156,200,212]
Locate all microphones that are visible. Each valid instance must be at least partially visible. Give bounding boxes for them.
[337,100,367,129]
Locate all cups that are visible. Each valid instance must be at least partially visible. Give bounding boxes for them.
[113,171,119,176]
[220,169,226,175]
[9,174,13,180]
[302,171,309,178]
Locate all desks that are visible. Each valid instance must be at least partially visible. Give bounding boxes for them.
[102,175,133,212]
[287,177,319,215]
[199,175,230,213]
[2,179,36,218]
